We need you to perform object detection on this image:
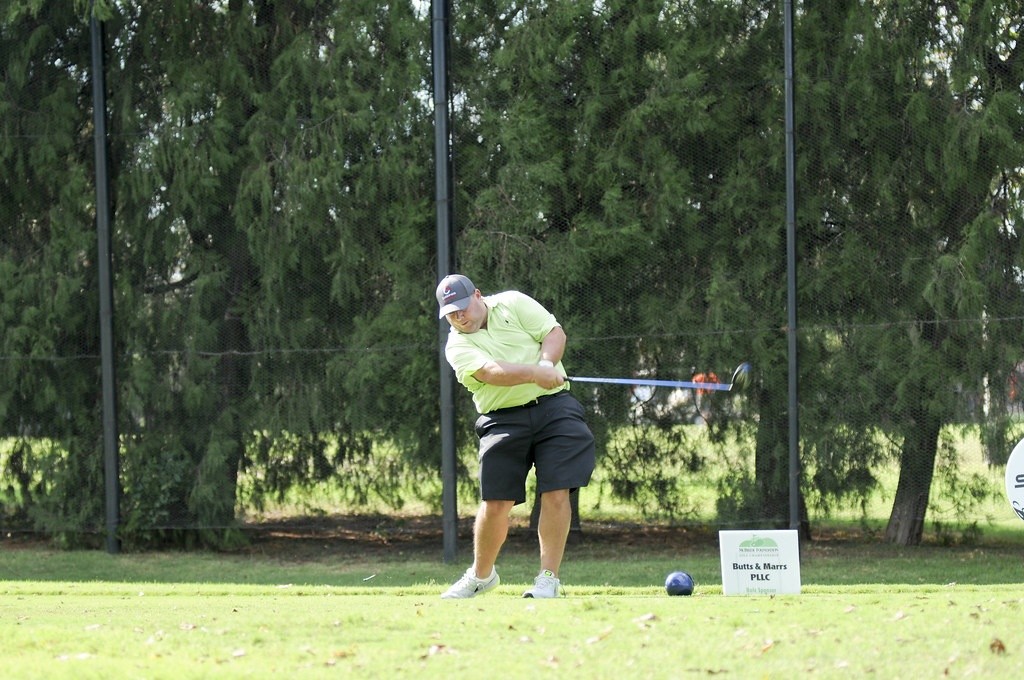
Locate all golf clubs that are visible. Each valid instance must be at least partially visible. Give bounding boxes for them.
[564,361,753,394]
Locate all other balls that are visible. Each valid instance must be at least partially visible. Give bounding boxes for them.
[665,570,694,596]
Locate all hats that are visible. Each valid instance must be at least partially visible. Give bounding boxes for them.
[436,274,476,319]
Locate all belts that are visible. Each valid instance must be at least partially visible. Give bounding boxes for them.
[489,394,553,414]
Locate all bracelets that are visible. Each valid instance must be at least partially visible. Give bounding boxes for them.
[538,361,553,366]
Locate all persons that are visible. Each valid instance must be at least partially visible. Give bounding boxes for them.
[693,372,719,419]
[436,274,594,597]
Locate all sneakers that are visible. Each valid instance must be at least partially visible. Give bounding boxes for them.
[520,570,566,599]
[441,564,500,599]
